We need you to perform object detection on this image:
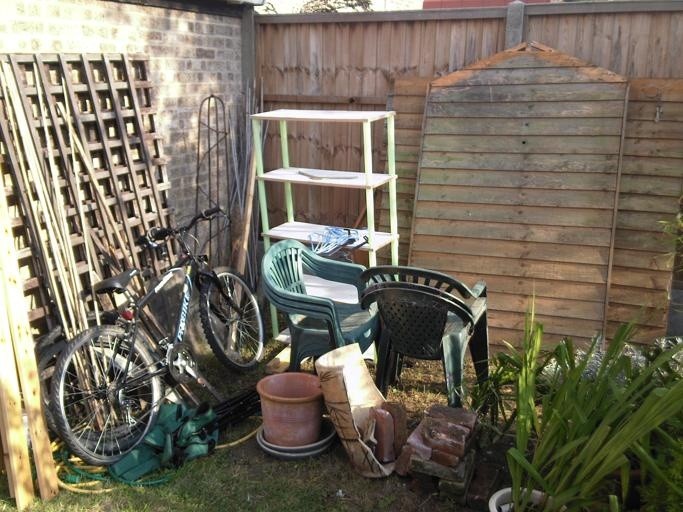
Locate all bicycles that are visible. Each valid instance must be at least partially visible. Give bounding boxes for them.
[51,206,265,467]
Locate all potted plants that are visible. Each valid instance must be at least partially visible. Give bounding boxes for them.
[488,280,683,512]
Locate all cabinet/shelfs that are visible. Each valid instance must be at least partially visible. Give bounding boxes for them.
[253,108,401,335]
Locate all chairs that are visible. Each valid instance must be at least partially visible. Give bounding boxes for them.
[261,240,490,409]
